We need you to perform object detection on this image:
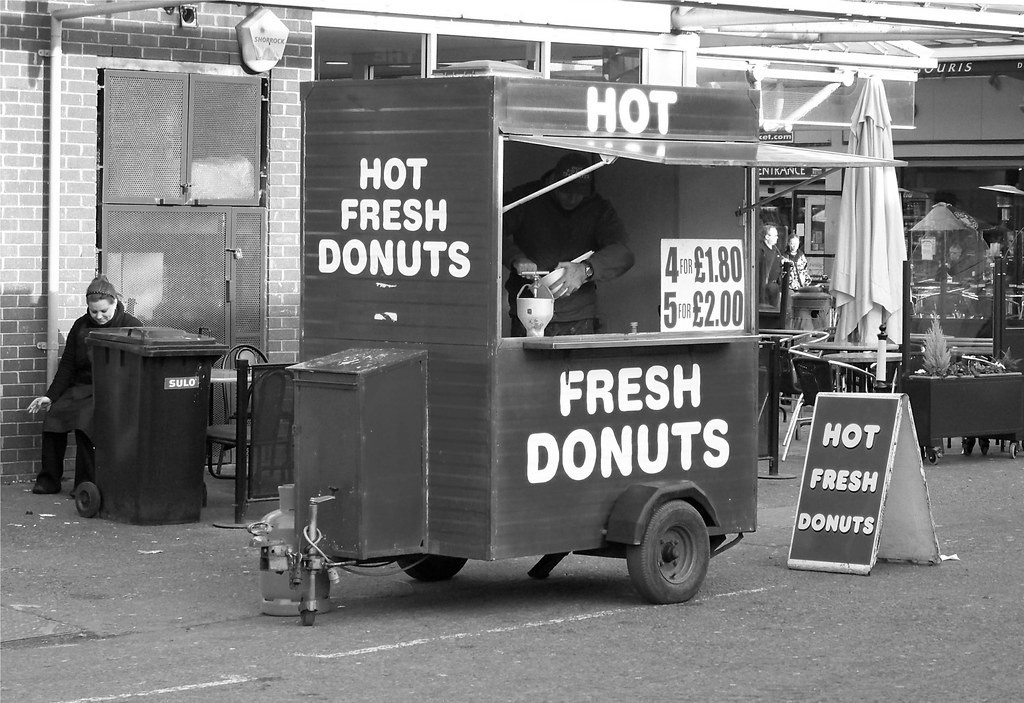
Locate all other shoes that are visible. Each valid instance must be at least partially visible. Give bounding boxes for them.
[69,488,78,497]
[33,482,63,495]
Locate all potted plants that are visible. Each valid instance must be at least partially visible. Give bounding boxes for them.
[904,304,1024,464]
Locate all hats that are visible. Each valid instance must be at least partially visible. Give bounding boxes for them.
[546,152,593,195]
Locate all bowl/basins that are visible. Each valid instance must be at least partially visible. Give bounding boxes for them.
[529,267,568,299]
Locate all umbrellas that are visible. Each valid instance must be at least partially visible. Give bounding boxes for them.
[828,75,908,347]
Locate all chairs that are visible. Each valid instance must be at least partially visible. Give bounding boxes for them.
[759,327,877,463]
[217,344,270,476]
[206,367,295,488]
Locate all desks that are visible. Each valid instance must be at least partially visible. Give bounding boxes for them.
[209,368,262,480]
[798,335,902,393]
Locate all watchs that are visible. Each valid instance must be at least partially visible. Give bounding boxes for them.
[582,260,593,280]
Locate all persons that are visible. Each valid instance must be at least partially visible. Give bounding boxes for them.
[26,275,143,498]
[502,154,635,337]
[758,224,812,308]
[936,244,971,282]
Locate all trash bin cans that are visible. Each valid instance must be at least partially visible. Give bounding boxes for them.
[75,327,229,527]
[790,293,830,334]
